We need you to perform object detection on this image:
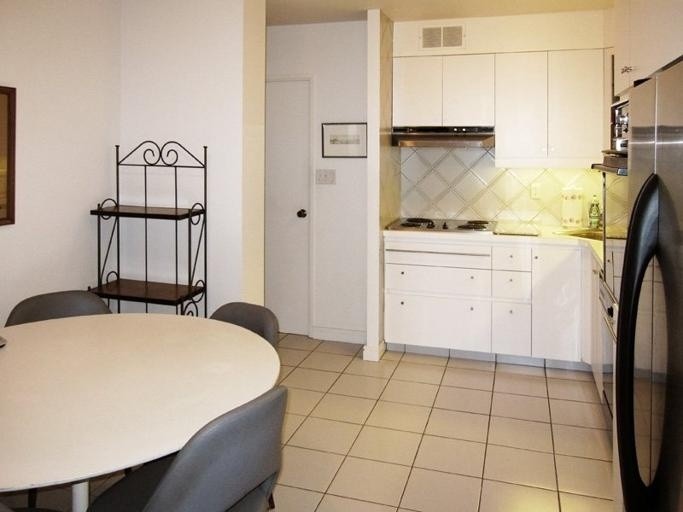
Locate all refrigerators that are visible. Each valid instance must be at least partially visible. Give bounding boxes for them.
[611,64,683,508]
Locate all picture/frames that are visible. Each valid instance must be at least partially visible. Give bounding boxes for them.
[0,86,16,225]
[321,122,367,158]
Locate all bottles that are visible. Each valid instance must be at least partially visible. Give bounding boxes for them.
[588,194,600,229]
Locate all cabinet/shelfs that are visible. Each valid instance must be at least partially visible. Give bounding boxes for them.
[583,239,604,402]
[383,231,492,361]
[392,53,495,129]
[606,241,655,379]
[532,237,582,370]
[653,252,683,386]
[491,236,532,368]
[495,49,605,167]
[89,140,207,314]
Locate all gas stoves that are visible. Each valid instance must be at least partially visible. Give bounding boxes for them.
[382,216,497,240]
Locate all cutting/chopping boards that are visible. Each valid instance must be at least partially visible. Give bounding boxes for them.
[493,219,541,236]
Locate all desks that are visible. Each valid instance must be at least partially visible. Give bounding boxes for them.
[0,314,280,512]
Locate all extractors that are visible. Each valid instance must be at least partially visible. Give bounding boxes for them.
[391,127,495,149]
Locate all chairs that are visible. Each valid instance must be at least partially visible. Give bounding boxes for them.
[87,386,288,512]
[208,304,280,348]
[5,291,133,511]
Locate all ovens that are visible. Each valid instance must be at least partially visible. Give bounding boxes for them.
[595,274,619,422]
